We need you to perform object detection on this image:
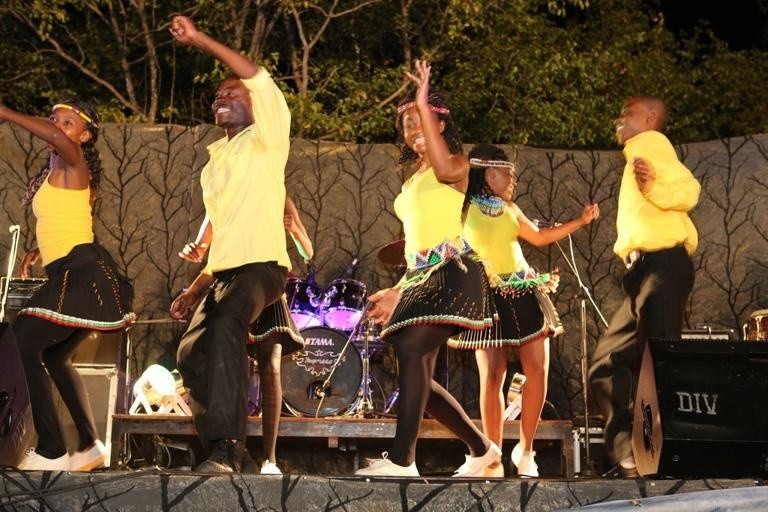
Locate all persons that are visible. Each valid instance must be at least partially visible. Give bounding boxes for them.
[586,93,700,479]
[459,143,599,479]
[0,95,137,471]
[170,15,292,474]
[177,196,305,475]
[354,60,503,477]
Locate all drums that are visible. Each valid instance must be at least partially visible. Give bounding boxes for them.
[324,278,370,331]
[280,324,365,415]
[284,277,320,327]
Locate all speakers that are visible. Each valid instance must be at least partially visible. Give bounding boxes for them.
[632,340,768,479]
[0,320,35,470]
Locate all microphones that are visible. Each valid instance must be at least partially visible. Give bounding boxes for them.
[532,218,563,228]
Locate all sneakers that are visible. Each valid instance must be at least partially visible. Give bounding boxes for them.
[16,441,106,471]
[355,457,420,479]
[452,441,540,478]
[194,460,234,473]
[260,458,282,475]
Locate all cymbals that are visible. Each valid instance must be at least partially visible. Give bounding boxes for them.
[379,238,408,265]
